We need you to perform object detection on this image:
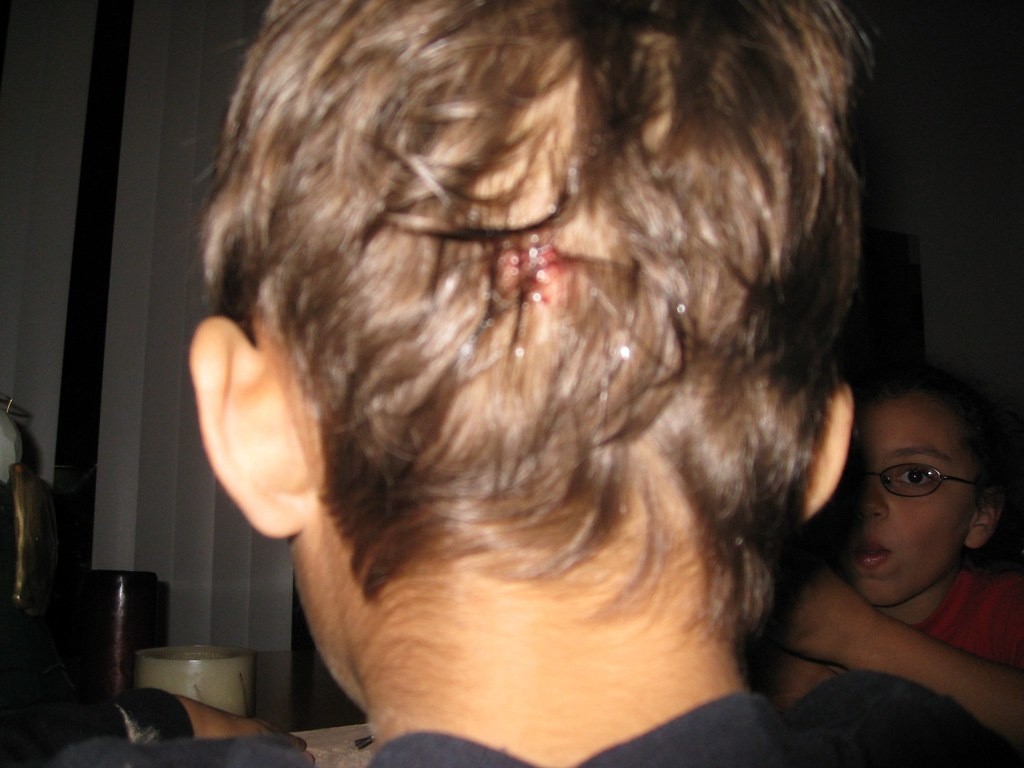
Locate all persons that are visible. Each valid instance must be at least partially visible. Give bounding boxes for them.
[759,361,1024,746]
[0,687,315,768]
[41,0,1024,767]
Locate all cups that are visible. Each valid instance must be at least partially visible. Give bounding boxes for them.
[83,569,159,706]
[133,645,255,718]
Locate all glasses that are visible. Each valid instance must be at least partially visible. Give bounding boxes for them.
[844,461,983,497]
